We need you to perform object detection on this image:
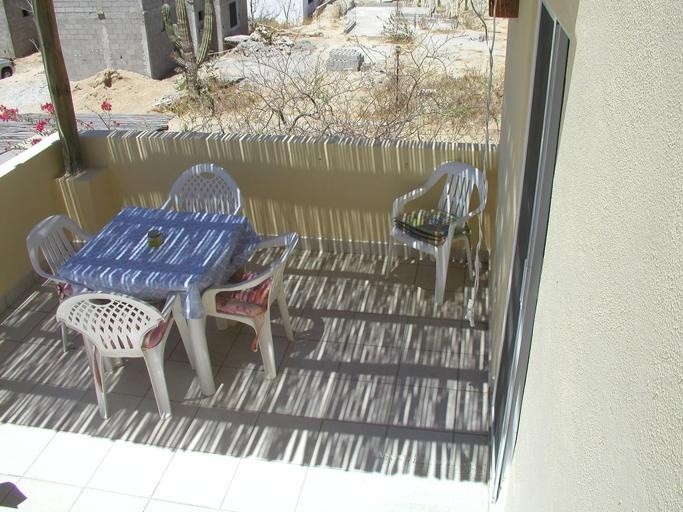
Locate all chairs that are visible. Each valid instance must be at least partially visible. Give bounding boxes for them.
[56,290,195,419]
[161,163,245,215]
[201,231,298,379]
[384,159,490,306]
[28,214,91,352]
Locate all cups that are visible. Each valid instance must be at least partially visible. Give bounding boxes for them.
[147,230,164,248]
